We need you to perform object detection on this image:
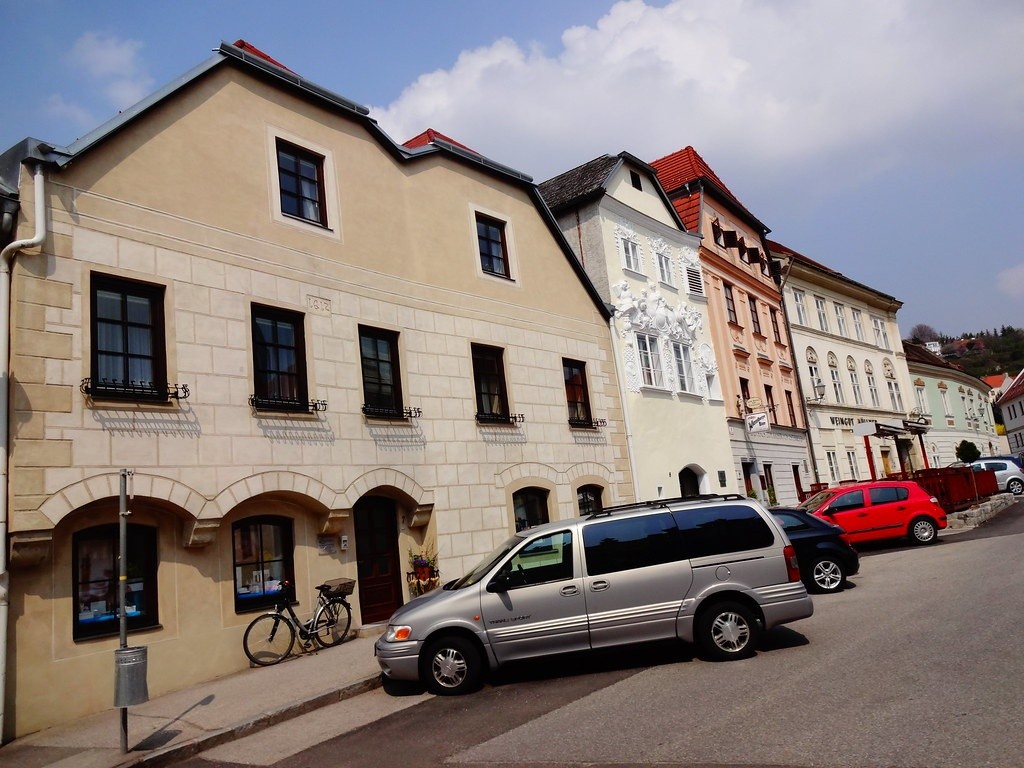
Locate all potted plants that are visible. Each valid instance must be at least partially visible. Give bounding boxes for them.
[767,485,780,506]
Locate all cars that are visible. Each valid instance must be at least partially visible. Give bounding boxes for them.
[948,454,1024,496]
[760,505,860,595]
[792,477,947,552]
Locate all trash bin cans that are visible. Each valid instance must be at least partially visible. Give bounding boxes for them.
[113,646,150,707]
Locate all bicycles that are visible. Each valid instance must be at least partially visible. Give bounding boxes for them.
[242,574,352,666]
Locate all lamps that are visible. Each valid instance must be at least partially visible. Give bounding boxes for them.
[971,405,985,418]
[805,378,827,417]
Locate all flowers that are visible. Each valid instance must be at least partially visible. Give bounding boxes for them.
[407,538,445,575]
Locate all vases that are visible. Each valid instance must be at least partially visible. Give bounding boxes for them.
[414,566,434,581]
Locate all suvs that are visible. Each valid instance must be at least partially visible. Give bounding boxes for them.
[377,495,814,699]
